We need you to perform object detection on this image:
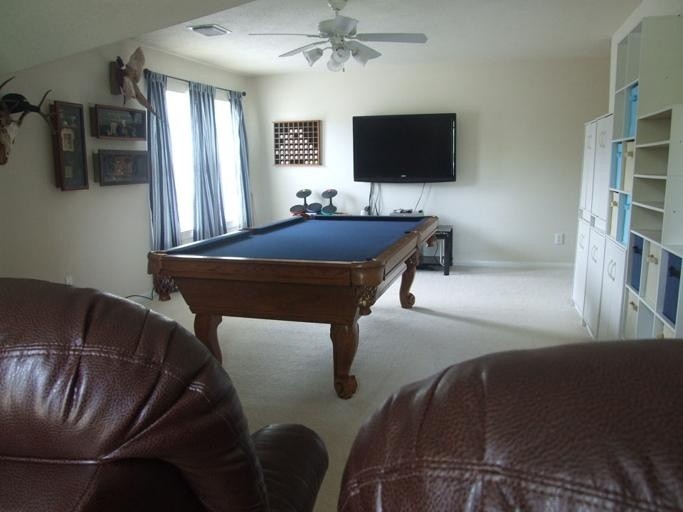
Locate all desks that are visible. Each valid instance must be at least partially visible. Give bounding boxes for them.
[147,212,440,399]
[414,224,454,275]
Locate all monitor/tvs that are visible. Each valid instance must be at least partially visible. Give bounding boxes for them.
[352,113,457,184]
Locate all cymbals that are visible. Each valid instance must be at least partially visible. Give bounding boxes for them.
[322,189,337,198]
[296,189,312,198]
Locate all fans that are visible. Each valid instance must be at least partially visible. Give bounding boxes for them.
[248,0,430,74]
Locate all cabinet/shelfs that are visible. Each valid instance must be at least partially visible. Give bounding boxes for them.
[570,13,683,343]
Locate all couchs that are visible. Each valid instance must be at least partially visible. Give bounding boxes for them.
[336,335,682,512]
[1,277,329,509]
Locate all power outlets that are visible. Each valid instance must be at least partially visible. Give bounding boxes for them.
[555,233,563,245]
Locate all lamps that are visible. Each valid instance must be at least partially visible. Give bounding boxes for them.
[303,0,365,73]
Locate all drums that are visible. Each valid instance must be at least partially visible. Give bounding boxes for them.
[321,205,337,215]
[306,203,322,213]
[290,205,304,214]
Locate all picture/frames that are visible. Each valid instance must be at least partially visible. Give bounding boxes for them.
[92,103,148,141]
[49,100,92,192]
[94,149,150,187]
